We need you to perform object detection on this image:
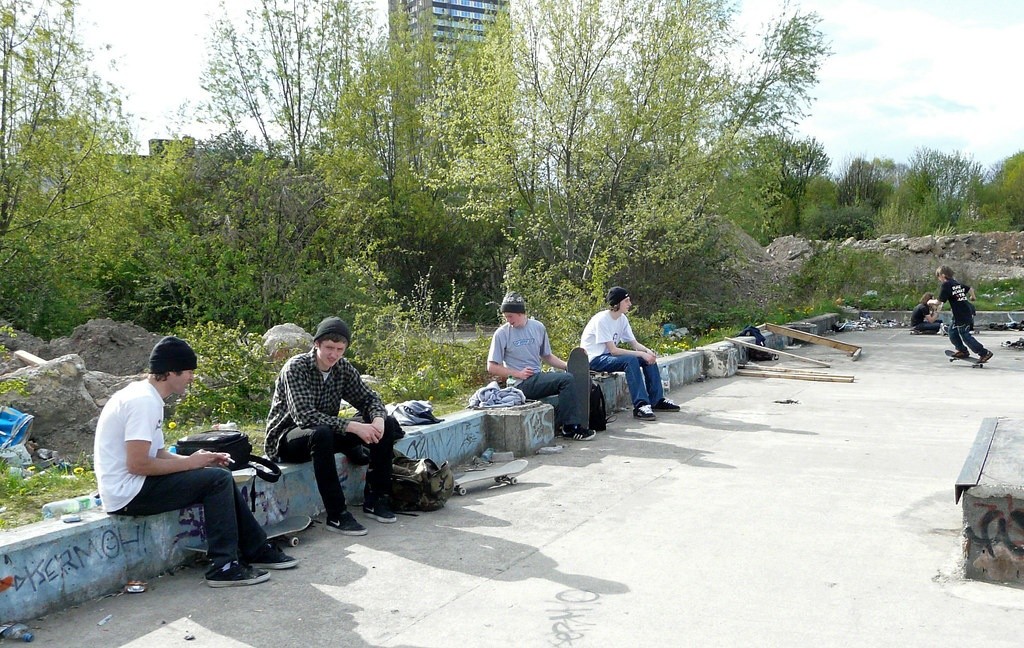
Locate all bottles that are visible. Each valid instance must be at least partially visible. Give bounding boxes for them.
[41,496,101,519]
[169,447,176,453]
[660,366,670,396]
[506,376,515,387]
[481,448,494,461]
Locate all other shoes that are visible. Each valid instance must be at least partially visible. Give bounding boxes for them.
[978,351,993,363]
[955,350,970,358]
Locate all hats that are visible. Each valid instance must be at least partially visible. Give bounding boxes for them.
[501,291,524,313]
[149,336,196,373]
[607,287,629,305]
[314,316,350,348]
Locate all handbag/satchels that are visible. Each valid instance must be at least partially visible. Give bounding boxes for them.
[588,384,607,431]
[389,456,454,512]
[175,429,281,483]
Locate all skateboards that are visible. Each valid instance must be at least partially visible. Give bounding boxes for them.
[944,349,988,368]
[566,347,590,431]
[183,515,312,553]
[452,458,529,495]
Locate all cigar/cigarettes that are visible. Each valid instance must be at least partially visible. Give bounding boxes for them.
[228,458,235,463]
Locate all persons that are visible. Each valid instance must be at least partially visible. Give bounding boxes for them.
[911,292,943,331]
[926,265,993,363]
[94,336,298,587]
[264,316,397,536]
[580,286,680,421]
[487,290,596,441]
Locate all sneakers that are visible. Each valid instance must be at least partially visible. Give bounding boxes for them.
[651,398,680,412]
[325,511,368,535]
[561,425,596,440]
[362,503,396,523]
[204,560,271,587]
[632,405,656,421]
[244,544,299,569]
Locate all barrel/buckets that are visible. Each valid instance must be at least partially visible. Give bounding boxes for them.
[0,407,35,452]
[664,324,673,335]
